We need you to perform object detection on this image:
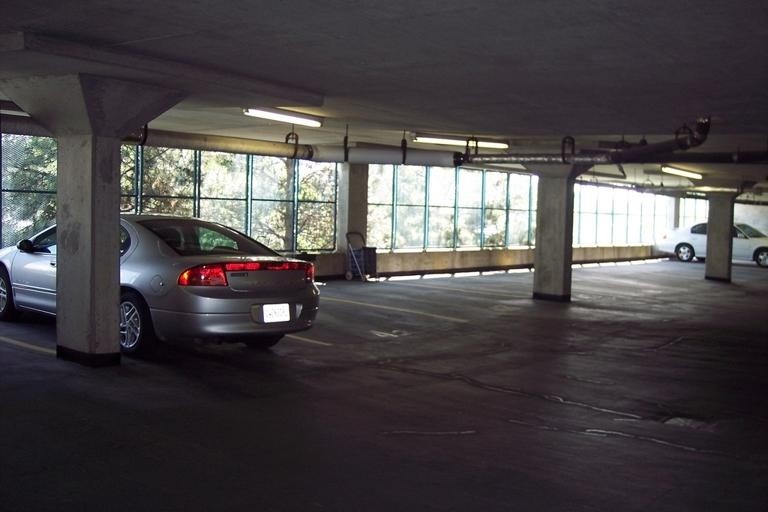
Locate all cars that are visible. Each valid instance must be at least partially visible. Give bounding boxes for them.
[657,222,767,268]
[0,211,319,356]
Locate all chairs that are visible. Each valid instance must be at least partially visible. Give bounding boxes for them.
[155,228,187,255]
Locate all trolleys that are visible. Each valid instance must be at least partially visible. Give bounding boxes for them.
[343,232,376,282]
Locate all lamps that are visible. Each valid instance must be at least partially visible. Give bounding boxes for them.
[660,165,703,182]
[243,105,324,129]
[412,133,510,150]
